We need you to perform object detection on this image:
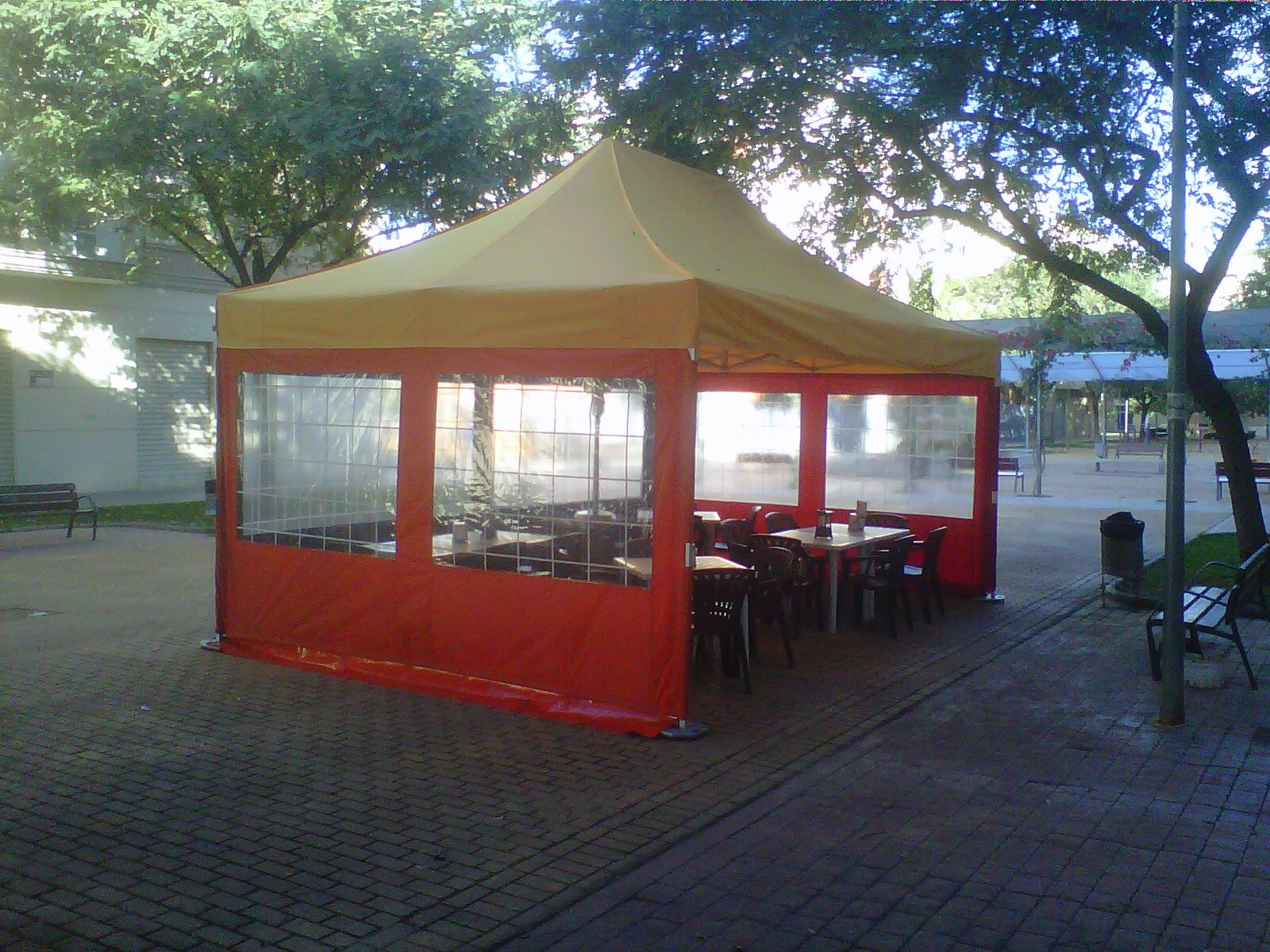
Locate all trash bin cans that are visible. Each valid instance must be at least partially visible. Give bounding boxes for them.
[1101,517,1146,578]
[1094,435,1108,457]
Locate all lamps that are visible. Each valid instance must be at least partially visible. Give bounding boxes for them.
[815,507,834,540]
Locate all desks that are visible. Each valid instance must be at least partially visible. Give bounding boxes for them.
[637,510,721,554]
[614,555,751,678]
[359,527,556,570]
[769,522,910,634]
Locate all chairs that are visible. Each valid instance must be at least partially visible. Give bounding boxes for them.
[716,506,828,669]
[549,510,650,583]
[840,513,948,639]
[689,567,756,695]
[324,542,377,558]
[692,516,705,557]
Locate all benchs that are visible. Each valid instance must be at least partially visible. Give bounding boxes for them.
[1114,440,1165,461]
[948,457,1025,495]
[1145,542,1270,692]
[0,484,98,541]
[1214,461,1270,501]
